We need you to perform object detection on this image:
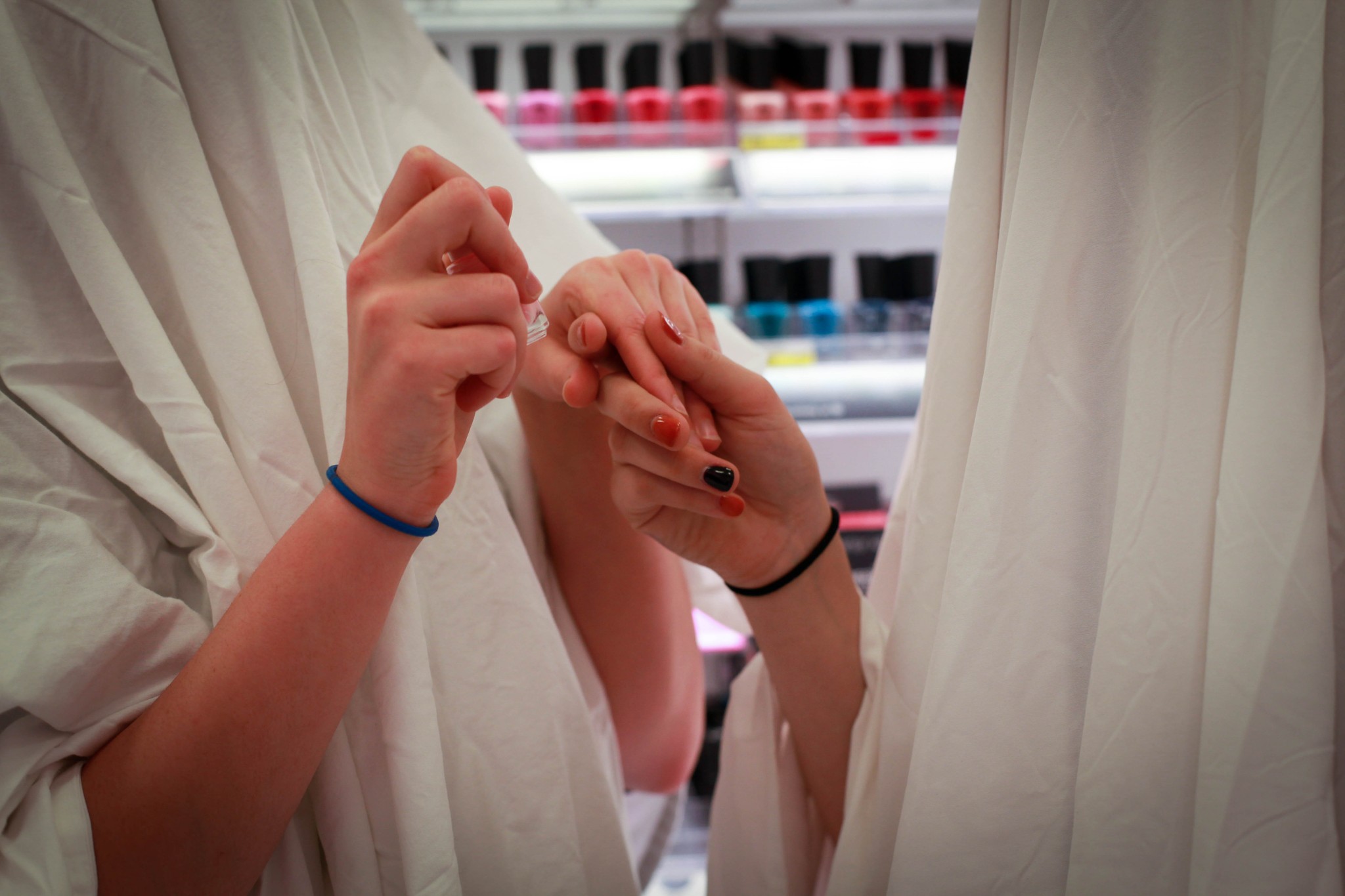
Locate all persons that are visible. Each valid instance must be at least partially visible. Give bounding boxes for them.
[2,0,774,896]
[565,3,1345,896]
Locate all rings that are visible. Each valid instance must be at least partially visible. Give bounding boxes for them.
[440,250,458,277]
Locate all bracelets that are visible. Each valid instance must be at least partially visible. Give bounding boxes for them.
[323,463,441,539]
[722,503,842,598]
